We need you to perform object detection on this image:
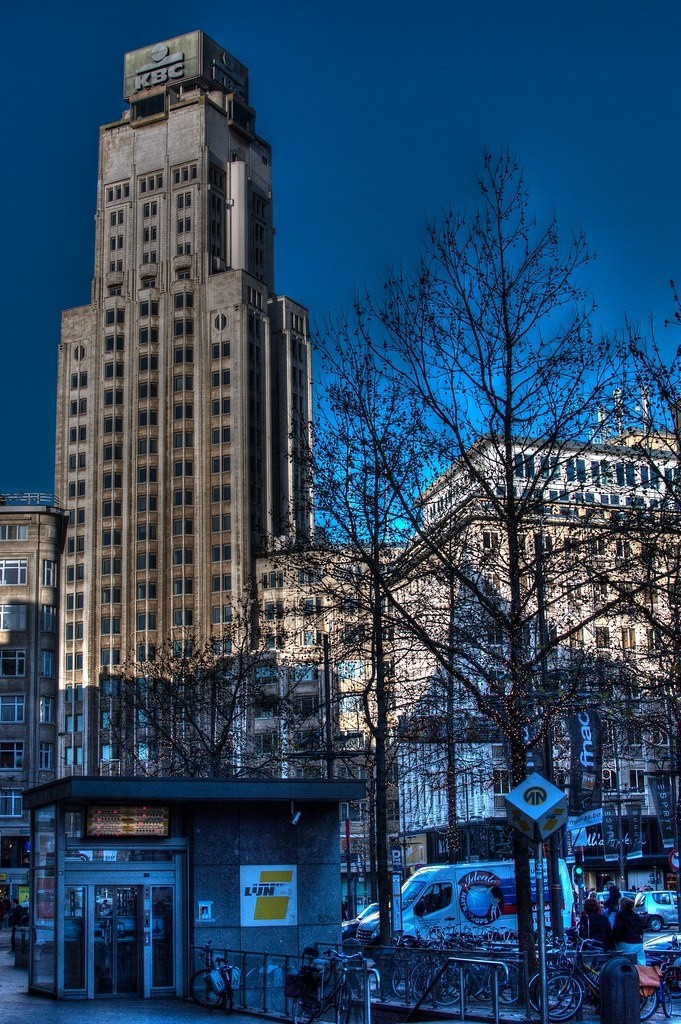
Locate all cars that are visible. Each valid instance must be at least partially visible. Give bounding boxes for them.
[595,891,637,908]
[633,890,680,931]
[342,899,422,944]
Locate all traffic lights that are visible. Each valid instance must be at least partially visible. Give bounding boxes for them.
[572,864,583,885]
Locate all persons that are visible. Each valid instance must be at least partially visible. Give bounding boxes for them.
[0,895,11,930]
[7,898,23,954]
[573,881,653,967]
[342,896,349,921]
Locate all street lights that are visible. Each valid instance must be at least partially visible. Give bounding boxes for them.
[298,732,363,779]
[646,755,670,776]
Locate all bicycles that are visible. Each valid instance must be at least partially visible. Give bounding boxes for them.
[293,949,363,1024]
[367,923,681,1022]
[191,938,236,1015]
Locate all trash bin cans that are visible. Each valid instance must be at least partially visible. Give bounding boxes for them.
[598,955,641,1024]
[14,926,30,969]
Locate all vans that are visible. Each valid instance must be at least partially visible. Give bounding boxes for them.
[355,860,577,942]
